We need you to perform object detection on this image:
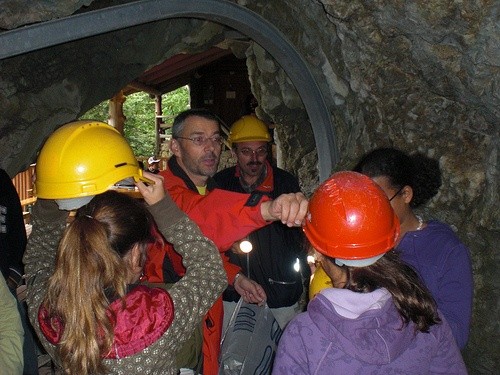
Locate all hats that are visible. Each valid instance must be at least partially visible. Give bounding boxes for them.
[148,157,161,164]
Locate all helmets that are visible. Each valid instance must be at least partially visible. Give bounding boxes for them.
[32,118,154,210]
[308,266,334,302]
[301,170,400,259]
[228,113,273,143]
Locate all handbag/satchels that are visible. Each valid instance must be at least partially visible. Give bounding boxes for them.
[218,295,282,375]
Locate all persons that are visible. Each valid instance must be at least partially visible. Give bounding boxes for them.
[0,109,473,375]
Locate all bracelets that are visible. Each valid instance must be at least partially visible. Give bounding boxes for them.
[234,273,240,280]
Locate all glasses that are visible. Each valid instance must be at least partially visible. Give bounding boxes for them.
[239,146,268,156]
[176,136,224,147]
